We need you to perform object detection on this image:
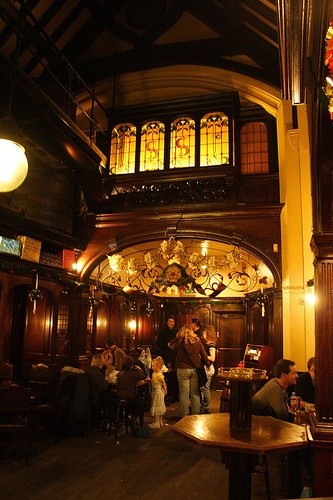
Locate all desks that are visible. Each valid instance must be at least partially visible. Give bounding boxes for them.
[170,411,308,499]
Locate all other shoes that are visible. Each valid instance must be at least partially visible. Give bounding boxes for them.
[147,422,164,428]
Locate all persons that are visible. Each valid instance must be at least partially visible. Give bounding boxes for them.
[149,356,167,429]
[168,325,211,419]
[197,325,218,412]
[157,315,179,403]
[85,340,147,428]
[190,318,203,340]
[248,358,306,424]
[292,356,315,413]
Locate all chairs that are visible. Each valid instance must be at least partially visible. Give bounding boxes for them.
[1,343,166,456]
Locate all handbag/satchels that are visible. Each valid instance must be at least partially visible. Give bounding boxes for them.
[196,362,208,388]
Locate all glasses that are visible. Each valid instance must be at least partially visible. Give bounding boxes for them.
[310,369,315,373]
[286,370,297,375]
